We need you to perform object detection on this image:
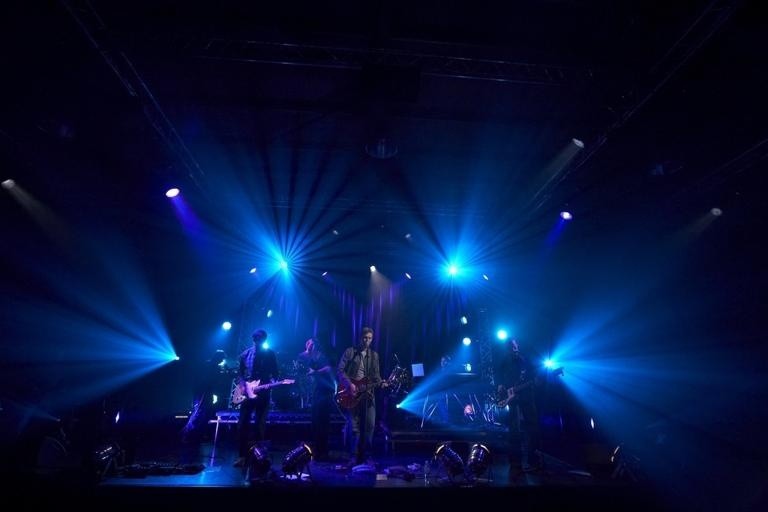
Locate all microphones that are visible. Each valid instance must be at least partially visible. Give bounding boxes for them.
[365,341,369,348]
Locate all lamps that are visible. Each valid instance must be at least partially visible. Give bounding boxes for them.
[280,444,313,479]
[96,442,121,473]
[468,443,491,478]
[434,443,464,482]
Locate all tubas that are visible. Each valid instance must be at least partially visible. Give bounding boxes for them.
[386,355,406,399]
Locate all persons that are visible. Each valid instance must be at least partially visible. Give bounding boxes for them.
[296,337,332,376]
[233,329,279,468]
[440,354,452,371]
[193,349,229,410]
[496,339,539,433]
[335,326,390,466]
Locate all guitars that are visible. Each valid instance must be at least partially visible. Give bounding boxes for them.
[232,377,295,403]
[496,366,563,410]
[335,370,409,409]
